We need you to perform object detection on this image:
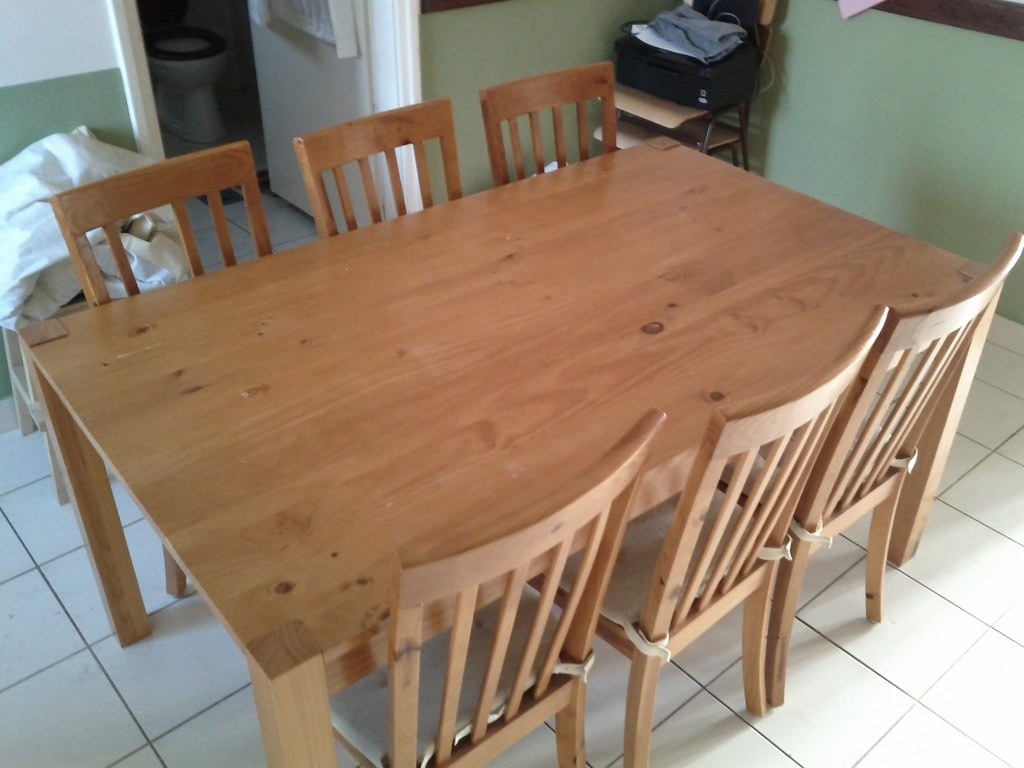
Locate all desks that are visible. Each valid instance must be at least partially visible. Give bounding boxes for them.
[25,136,996,768]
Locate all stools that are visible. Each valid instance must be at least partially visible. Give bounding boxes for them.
[2,301,87,507]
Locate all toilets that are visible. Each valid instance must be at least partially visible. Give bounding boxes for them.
[136,0,231,145]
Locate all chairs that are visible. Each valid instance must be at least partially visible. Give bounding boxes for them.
[717,228,1024,707]
[479,0,778,188]
[331,409,667,768]
[526,306,887,768]
[51,141,272,306]
[291,98,463,237]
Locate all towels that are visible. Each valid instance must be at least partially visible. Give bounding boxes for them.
[633,4,748,65]
